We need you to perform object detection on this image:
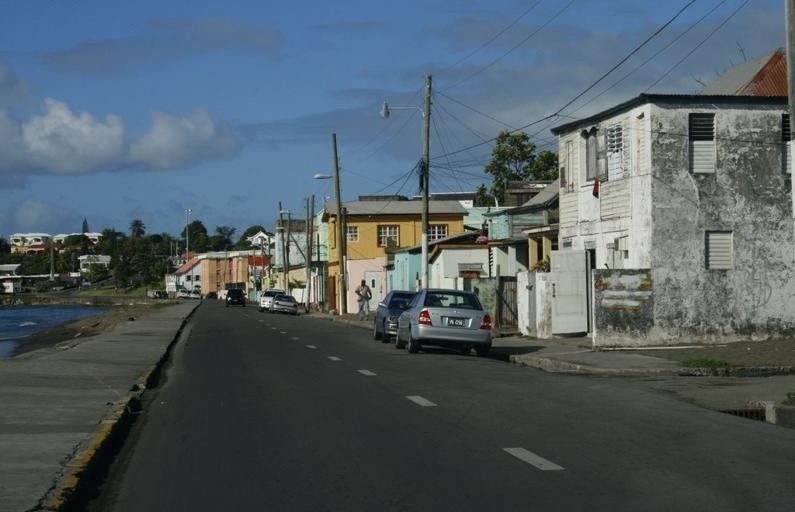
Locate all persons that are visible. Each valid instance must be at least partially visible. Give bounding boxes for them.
[355,280,372,321]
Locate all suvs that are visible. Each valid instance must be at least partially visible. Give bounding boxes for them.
[258,288,286,313]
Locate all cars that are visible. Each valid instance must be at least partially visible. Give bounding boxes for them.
[268,293,298,316]
[224,288,248,307]
[146,284,201,299]
[372,289,446,343]
[393,287,494,356]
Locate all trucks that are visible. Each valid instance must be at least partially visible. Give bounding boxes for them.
[64,278,91,289]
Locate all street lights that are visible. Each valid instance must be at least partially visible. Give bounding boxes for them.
[278,209,314,308]
[184,205,194,264]
[380,100,431,290]
[313,173,347,314]
